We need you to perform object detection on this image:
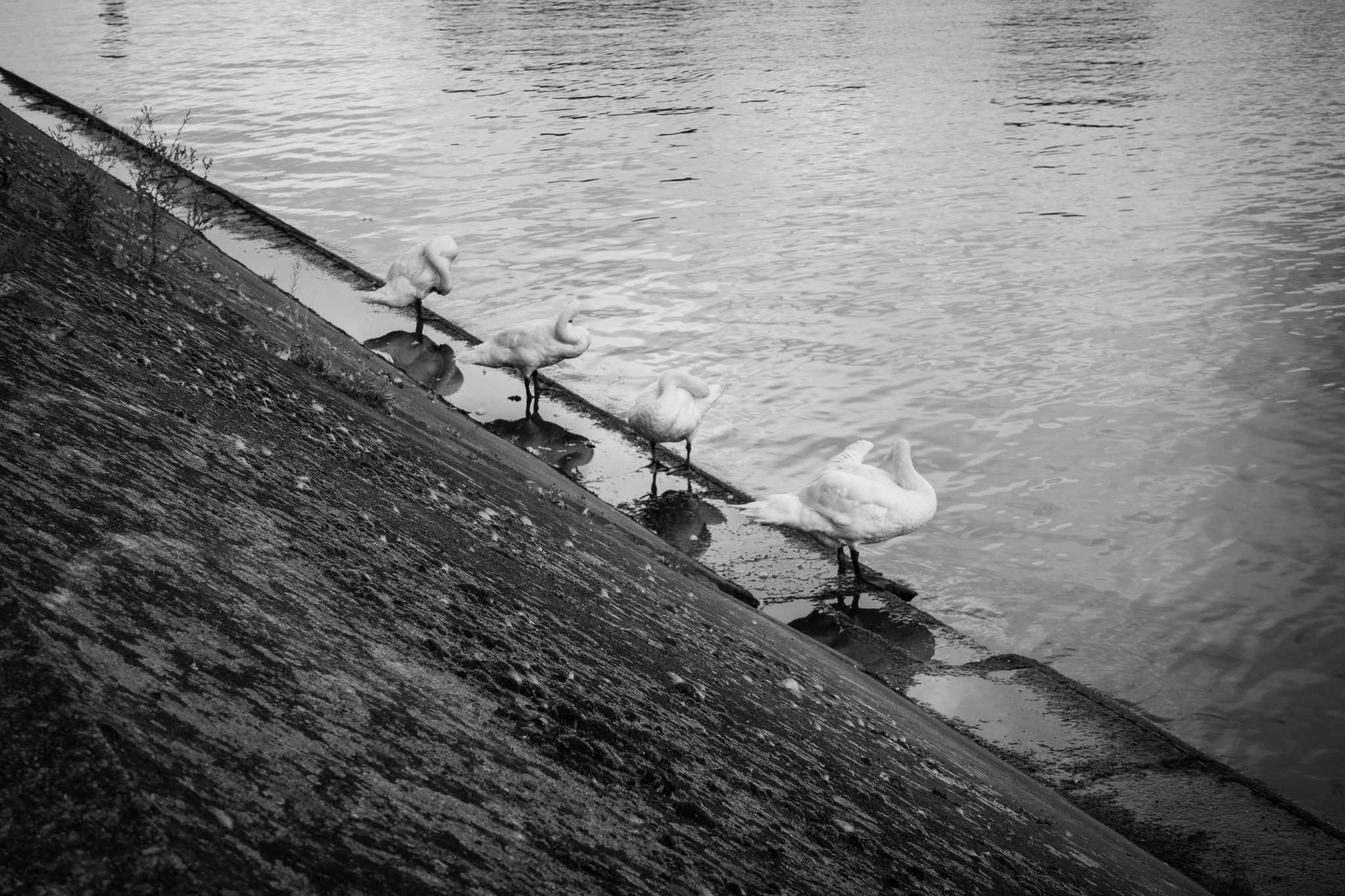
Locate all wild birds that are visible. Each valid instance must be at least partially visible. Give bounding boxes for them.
[724,434,939,594]
[448,296,594,404]
[353,232,460,323]
[627,363,731,479]
